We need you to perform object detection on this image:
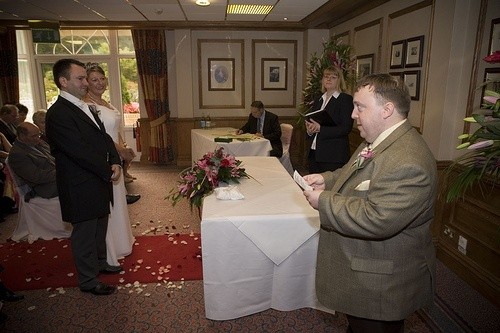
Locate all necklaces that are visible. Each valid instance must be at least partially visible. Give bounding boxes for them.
[88,93,105,105]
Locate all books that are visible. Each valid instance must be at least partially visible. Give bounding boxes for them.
[296,109,336,127]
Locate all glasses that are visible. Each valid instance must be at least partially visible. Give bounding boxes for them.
[23,134,42,141]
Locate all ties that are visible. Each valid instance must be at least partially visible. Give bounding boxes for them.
[256,119,261,134]
[352,144,372,168]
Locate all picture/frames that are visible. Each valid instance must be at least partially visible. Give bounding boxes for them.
[487,17,500,56]
[261,58,287,91]
[480,67,500,110]
[390,35,425,101]
[208,58,235,91]
[356,53,374,80]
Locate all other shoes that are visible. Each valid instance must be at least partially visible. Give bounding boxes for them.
[125,178,133,183]
[124,173,136,180]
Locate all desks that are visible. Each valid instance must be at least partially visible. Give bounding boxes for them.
[201,157,320,321]
[191,127,273,169]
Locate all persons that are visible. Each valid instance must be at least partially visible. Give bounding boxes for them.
[0,102,141,204]
[236,101,283,159]
[43,58,122,295]
[300,72,441,333]
[304,65,354,175]
[81,61,136,267]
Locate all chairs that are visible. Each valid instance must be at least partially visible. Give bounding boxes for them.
[277,123,295,177]
[5,157,73,244]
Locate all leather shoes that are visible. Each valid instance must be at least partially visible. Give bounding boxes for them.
[101,265,122,274]
[80,282,114,295]
[126,194,140,204]
[0,289,24,301]
[0,312,7,322]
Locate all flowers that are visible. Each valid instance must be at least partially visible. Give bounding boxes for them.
[295,35,357,129]
[163,147,259,220]
[437,51,500,203]
